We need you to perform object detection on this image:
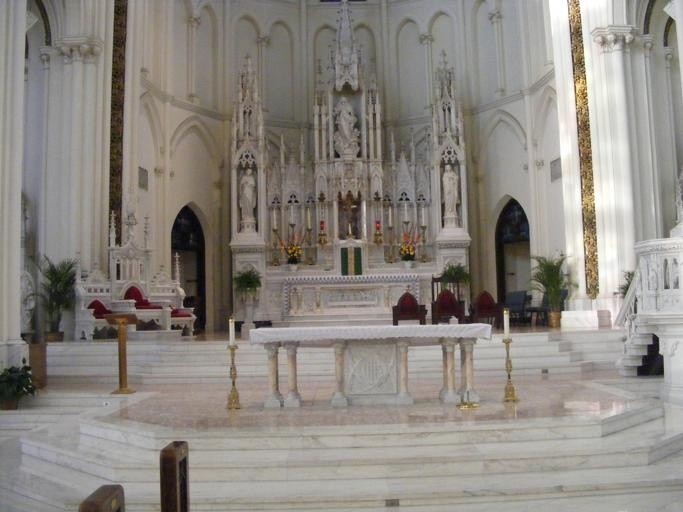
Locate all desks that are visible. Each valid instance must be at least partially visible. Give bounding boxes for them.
[248,320,489,409]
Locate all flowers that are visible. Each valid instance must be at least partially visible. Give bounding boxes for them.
[398,242,416,261]
[279,241,303,264]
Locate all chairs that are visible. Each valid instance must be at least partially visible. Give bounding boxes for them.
[107,208,172,336]
[429,271,459,304]
[146,247,196,342]
[504,289,528,326]
[527,287,571,331]
[430,288,465,326]
[467,287,505,333]
[73,259,136,345]
[390,290,429,325]
[158,440,190,511]
[73,480,131,511]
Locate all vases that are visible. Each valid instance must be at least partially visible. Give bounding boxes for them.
[288,263,298,272]
[403,259,413,270]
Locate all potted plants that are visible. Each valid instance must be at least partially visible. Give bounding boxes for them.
[0,357,39,412]
[24,256,76,347]
[528,251,580,328]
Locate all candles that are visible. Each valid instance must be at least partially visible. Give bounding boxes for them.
[404,200,407,223]
[348,222,351,235]
[227,314,236,347]
[290,205,294,226]
[502,306,512,340]
[388,204,392,227]
[272,205,277,230]
[306,208,311,230]
[419,207,425,227]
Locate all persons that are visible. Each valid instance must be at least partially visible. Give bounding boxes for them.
[237,167,258,222]
[334,95,360,157]
[442,165,460,229]
